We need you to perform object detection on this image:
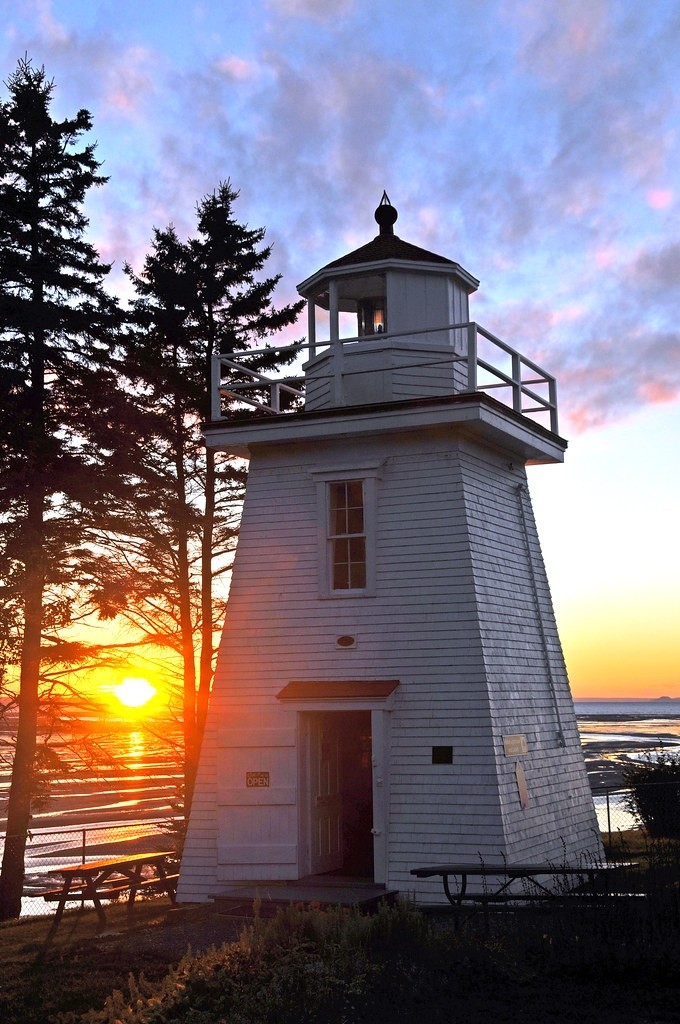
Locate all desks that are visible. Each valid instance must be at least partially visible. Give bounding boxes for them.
[410,863,648,944]
[46,851,183,933]
